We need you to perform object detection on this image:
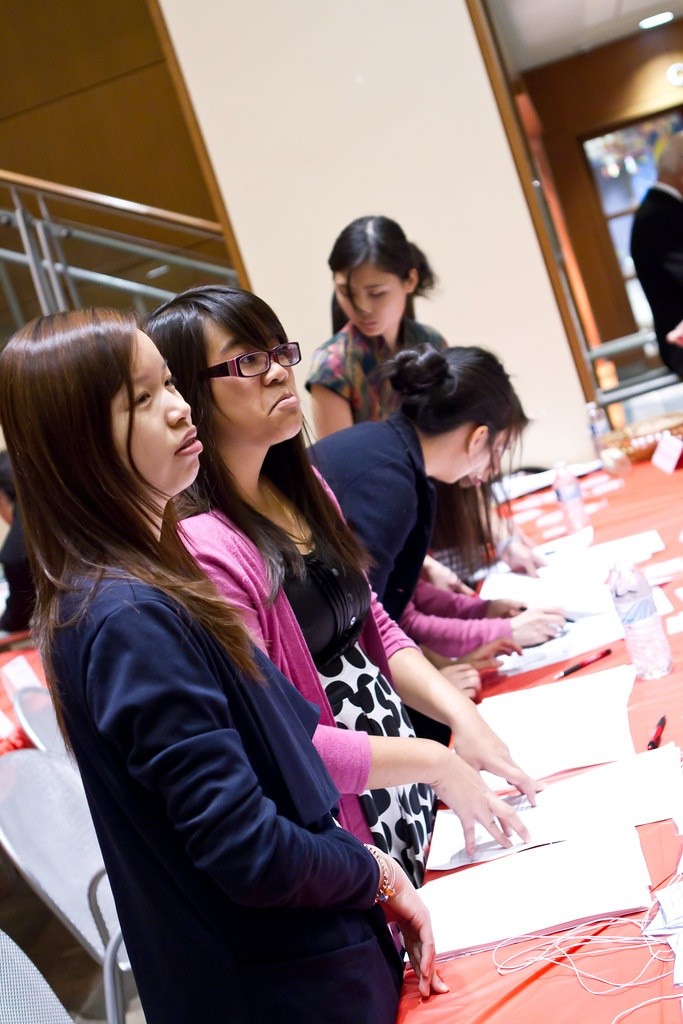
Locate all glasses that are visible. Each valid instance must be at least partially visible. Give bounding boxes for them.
[205,342,302,379]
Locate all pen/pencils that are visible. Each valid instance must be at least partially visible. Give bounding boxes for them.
[520,606,575,623]
[647,715,666,750]
[554,648,611,681]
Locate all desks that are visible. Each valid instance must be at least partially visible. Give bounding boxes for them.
[400,457,683,1024]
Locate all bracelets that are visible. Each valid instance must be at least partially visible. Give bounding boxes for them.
[365,843,390,904]
[374,845,396,902]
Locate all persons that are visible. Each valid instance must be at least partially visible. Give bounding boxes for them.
[0,306,449,1024]
[629,130,683,383]
[302,214,548,601]
[0,450,39,757]
[306,341,574,744]
[138,282,542,956]
